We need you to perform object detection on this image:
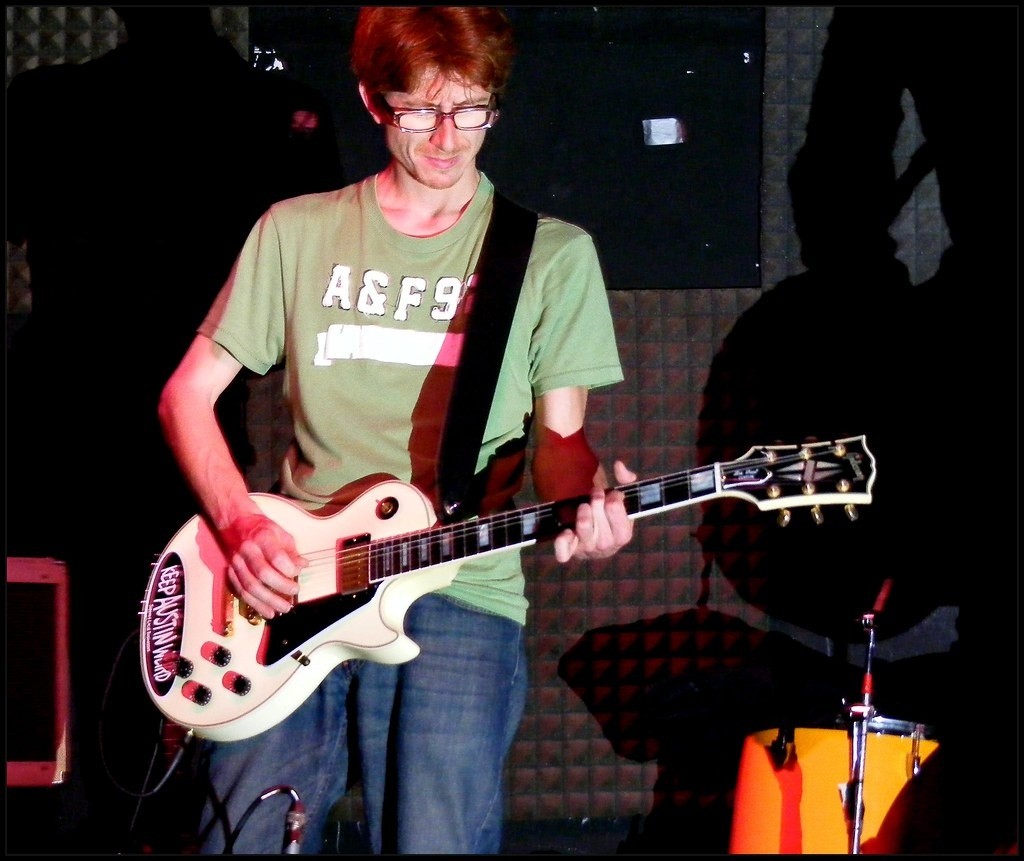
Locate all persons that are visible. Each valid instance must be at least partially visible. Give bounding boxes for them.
[158,8,637,854]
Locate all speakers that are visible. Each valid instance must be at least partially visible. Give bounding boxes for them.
[4,556,72,787]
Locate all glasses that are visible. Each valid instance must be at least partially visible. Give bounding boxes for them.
[374,89,500,134]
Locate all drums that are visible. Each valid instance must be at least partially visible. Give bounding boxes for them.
[728,709,941,856]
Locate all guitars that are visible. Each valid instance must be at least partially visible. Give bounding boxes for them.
[136,433,878,742]
